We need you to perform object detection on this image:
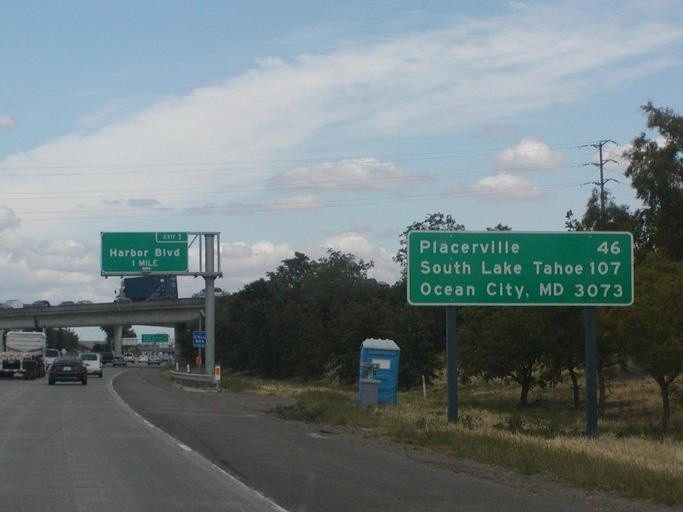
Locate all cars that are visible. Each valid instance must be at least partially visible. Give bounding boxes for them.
[1,299,94,310]
[46,348,104,386]
[102,351,169,367]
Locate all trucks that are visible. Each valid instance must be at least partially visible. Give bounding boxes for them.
[114,273,178,302]
[0,327,48,381]
